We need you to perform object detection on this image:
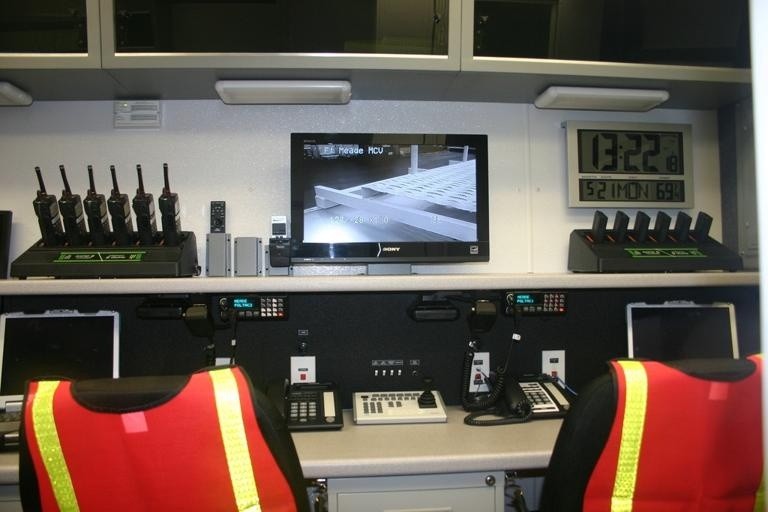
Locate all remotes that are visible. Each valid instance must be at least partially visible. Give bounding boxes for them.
[211,200,226,233]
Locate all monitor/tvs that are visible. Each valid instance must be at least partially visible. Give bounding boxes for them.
[0,309,121,413]
[625,299,741,363]
[289,131,490,277]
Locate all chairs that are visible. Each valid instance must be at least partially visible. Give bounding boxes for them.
[19,366,313,512]
[540,354,768,512]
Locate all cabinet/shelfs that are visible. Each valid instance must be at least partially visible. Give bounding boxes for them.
[0,1,752,112]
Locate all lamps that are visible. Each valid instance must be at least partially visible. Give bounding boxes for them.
[215,79,353,105]
[534,85,670,112]
[0,81,33,107]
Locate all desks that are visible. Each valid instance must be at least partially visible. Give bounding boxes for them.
[0,404,567,512]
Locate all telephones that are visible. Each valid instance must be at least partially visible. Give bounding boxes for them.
[485,372,573,419]
[264,378,343,431]
